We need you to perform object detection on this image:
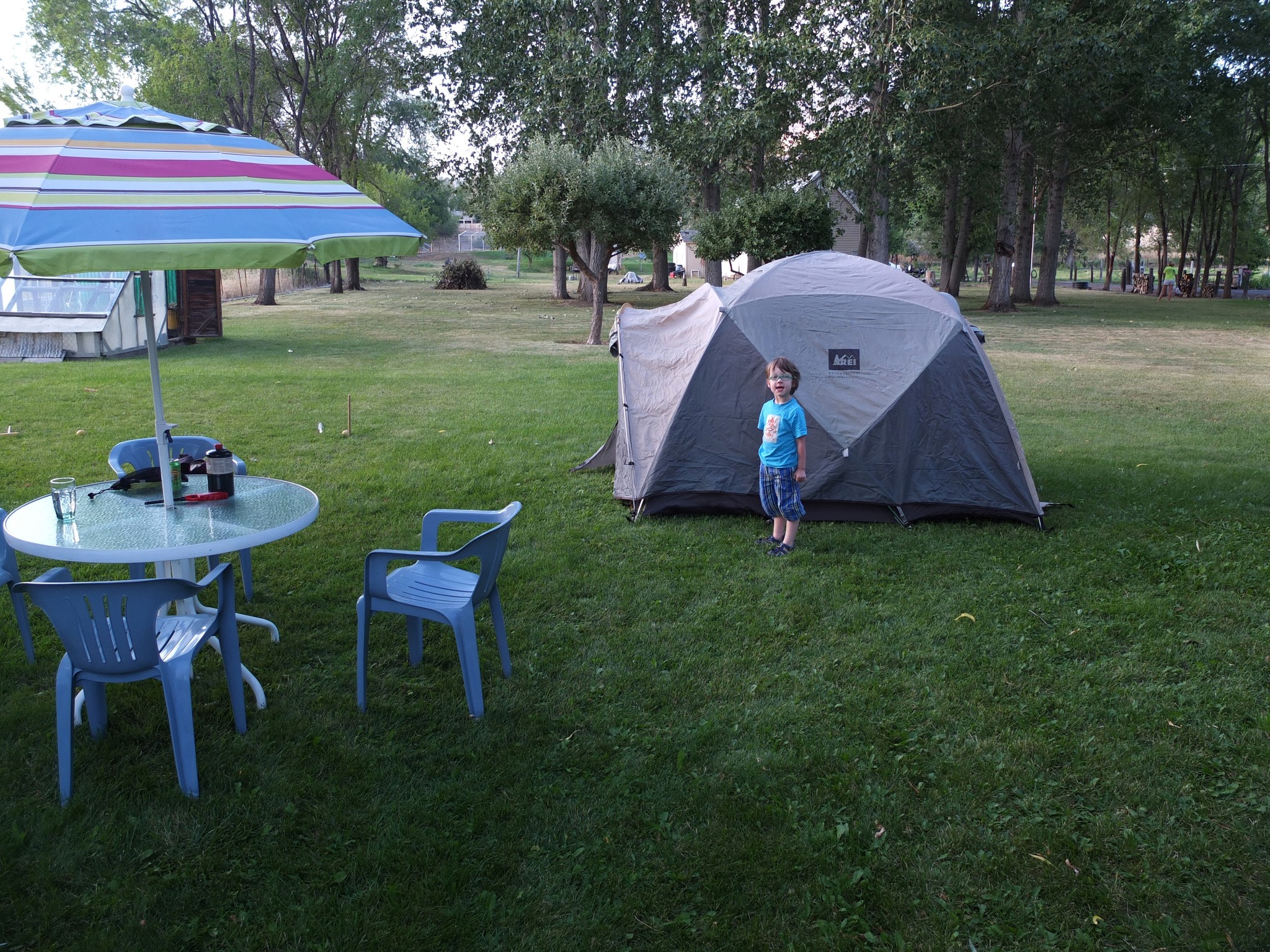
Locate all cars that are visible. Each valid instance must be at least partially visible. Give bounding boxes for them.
[889,262,902,272]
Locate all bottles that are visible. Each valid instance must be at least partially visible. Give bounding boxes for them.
[205,444,235,496]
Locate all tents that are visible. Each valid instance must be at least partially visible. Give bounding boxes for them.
[573,248,1052,536]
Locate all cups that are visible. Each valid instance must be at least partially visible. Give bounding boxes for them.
[50,477,77,520]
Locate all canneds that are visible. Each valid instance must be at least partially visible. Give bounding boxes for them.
[169,458,182,494]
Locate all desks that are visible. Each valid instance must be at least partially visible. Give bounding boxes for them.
[2,473,320,728]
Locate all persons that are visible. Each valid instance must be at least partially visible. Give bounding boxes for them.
[1156,261,1176,303]
[754,357,807,559]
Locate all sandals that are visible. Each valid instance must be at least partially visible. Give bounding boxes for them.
[767,541,794,557]
[755,535,783,545]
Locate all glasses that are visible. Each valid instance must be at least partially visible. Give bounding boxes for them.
[768,374,793,381]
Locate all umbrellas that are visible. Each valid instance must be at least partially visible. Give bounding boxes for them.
[0,82,428,509]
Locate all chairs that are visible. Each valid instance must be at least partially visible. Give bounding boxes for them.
[0,507,248,808]
[356,501,522,718]
[108,435,253,603]
